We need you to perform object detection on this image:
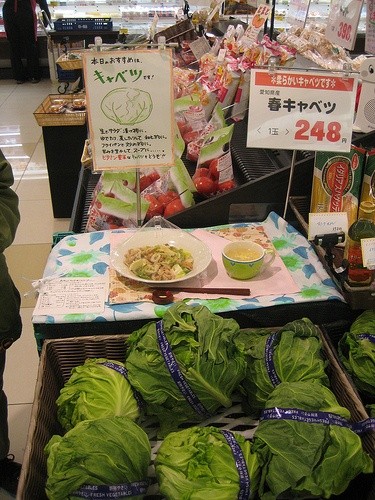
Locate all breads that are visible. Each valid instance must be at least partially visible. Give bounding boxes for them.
[49,98,86,112]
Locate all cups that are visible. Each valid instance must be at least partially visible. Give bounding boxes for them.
[222,241,275,280]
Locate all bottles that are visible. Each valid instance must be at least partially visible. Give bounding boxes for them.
[210,0,219,21]
[344,200,375,288]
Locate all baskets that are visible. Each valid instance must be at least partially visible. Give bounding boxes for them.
[55,50,83,70]
[33,93,86,126]
[53,17,113,31]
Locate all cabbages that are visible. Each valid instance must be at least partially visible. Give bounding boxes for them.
[44,302,375,500]
[129,244,193,280]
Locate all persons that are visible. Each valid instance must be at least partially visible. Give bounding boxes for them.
[0,149,23,494]
[2,0,51,83]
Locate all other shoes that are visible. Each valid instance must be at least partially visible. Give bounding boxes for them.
[31,76,40,82]
[16,77,28,84]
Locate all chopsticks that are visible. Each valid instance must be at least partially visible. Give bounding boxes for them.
[150,286,251,295]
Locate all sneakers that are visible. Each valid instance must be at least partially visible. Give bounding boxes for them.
[0,454,22,497]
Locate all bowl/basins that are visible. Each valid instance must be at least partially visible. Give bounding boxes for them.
[111,231,212,284]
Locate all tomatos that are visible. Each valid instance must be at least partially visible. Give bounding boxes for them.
[93,122,233,230]
